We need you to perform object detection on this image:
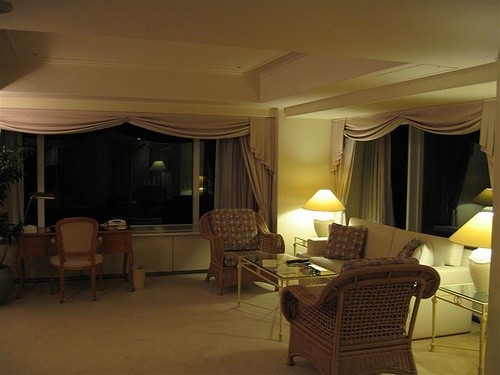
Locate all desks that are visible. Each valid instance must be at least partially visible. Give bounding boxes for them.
[16,229,135,297]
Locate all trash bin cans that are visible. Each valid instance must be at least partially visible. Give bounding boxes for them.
[132,265,146,290]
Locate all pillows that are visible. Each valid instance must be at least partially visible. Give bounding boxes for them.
[397,237,423,256]
[411,241,435,266]
[324,223,368,260]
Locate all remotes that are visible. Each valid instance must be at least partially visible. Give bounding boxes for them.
[288,261,311,267]
[285,258,309,264]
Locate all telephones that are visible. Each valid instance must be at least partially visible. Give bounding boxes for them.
[108,219,126,226]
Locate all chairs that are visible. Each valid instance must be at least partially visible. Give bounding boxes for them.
[200,208,286,297]
[279,258,441,375]
[47,217,104,300]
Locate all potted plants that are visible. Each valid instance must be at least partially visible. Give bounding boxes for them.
[0,140,33,306]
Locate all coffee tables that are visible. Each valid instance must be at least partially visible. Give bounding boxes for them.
[236,252,339,342]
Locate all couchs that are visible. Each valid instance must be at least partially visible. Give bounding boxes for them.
[296,217,474,340]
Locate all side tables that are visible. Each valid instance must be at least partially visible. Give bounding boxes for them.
[429,282,489,375]
[292,237,319,256]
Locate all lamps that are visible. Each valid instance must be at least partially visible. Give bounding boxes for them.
[473,189,493,207]
[148,160,167,185]
[450,212,493,292]
[21,192,55,225]
[302,189,347,237]
